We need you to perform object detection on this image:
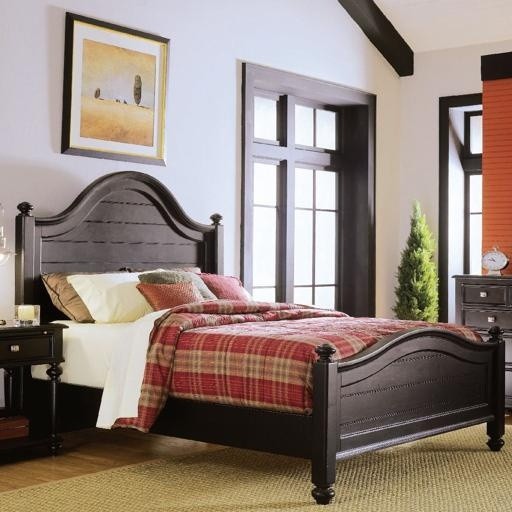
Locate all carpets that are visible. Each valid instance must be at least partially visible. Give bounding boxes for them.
[1,422,512,512]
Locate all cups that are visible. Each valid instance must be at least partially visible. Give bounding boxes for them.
[10,304,40,328]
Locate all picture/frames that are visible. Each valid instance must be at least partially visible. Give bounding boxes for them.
[60,10,172,167]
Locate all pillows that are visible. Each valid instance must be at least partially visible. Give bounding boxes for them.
[36,264,258,326]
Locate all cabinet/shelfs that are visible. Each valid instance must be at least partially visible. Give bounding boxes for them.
[448,276,511,419]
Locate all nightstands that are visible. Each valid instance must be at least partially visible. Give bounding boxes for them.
[1,327,69,460]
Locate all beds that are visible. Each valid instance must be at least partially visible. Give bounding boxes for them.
[11,172,508,506]
